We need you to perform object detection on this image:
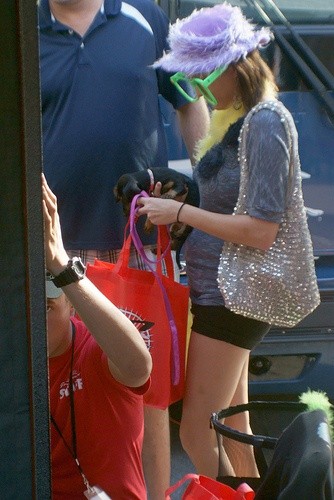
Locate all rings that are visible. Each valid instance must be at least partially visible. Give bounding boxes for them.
[152,192,161,196]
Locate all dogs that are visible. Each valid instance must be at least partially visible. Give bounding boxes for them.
[113,166,200,270]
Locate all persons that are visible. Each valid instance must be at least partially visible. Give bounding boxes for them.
[37,0,211,499]
[139,3,321,481]
[40,174,153,500]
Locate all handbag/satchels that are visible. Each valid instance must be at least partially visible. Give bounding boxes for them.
[74,205,190,410]
[208,401,334,500]
[164,473,256,500]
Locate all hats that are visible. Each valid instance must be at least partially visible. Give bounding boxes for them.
[46,280,63,299]
[147,0,275,78]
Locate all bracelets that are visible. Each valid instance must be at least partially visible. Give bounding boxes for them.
[177,201,184,223]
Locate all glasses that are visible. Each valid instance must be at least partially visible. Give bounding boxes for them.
[169,64,226,105]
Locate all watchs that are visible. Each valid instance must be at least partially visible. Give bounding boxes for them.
[52,256,86,288]
